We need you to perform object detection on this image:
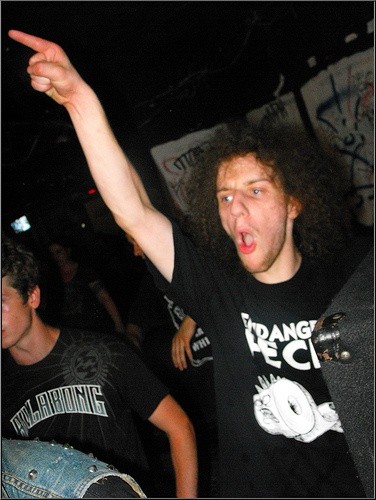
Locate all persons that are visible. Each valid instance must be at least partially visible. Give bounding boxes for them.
[8,28,374,498]
[123,231,215,414]
[42,240,125,337]
[0,241,199,498]
